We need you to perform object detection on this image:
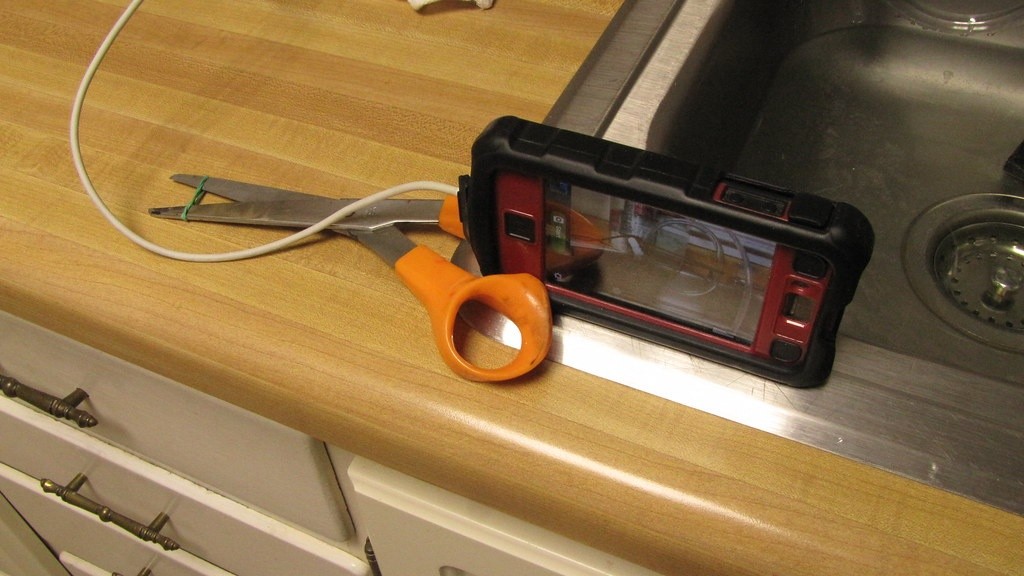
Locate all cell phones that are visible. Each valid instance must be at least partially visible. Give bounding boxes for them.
[456,115,875,388]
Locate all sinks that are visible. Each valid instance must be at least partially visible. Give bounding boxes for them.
[447,1,1024,518]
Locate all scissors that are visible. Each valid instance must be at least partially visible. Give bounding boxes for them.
[149,173,607,386]
